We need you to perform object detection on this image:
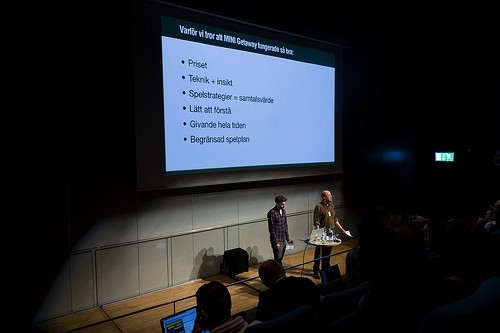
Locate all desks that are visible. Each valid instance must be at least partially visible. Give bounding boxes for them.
[298,236,341,276]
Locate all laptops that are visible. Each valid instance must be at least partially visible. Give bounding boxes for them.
[298,228,324,242]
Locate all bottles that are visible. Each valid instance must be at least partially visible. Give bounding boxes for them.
[316,229,337,244]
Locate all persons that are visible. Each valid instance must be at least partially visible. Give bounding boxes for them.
[469,201,496,240]
[266,194,295,266]
[254,257,322,325]
[190,282,248,333]
[311,190,357,279]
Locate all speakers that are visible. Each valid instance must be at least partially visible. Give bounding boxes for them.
[224,248,249,275]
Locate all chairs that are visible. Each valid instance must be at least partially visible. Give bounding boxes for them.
[244,273,500,333]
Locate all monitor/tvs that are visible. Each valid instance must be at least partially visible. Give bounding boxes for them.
[160,305,197,333]
[435,151,455,163]
